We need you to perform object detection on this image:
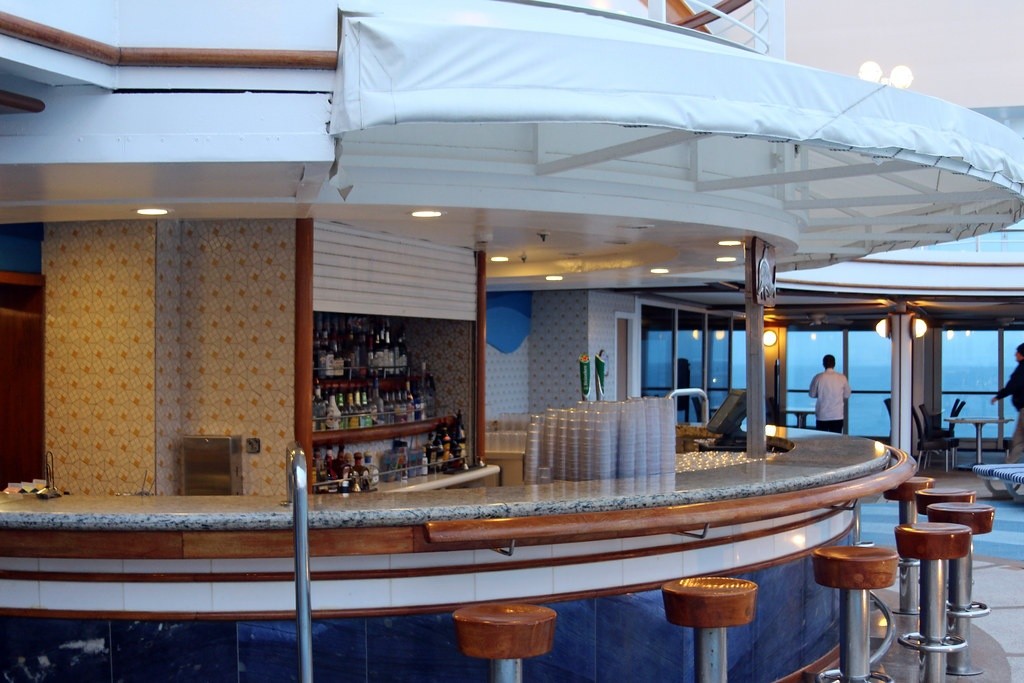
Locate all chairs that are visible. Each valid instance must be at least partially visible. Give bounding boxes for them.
[912,396,966,466]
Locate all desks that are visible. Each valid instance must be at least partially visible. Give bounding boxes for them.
[781,406,816,429]
[944,417,1011,470]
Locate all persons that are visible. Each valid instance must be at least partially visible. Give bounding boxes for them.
[809,355,851,433]
[991,343,1024,464]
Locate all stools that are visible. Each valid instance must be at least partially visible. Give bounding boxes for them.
[883,474,937,616]
[914,488,978,617]
[661,575,760,683]
[808,543,899,683]
[917,504,996,675]
[452,602,557,681]
[893,521,972,682]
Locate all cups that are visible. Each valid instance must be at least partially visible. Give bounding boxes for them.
[486,395,676,484]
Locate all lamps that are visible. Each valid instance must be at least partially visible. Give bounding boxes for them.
[763,328,777,347]
[910,319,927,340]
[875,318,891,339]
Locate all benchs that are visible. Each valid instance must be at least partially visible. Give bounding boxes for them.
[972,460,1024,502]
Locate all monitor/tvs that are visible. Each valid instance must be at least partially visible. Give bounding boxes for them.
[707,389,747,434]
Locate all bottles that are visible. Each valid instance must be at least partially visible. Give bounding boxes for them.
[313,312,485,493]
